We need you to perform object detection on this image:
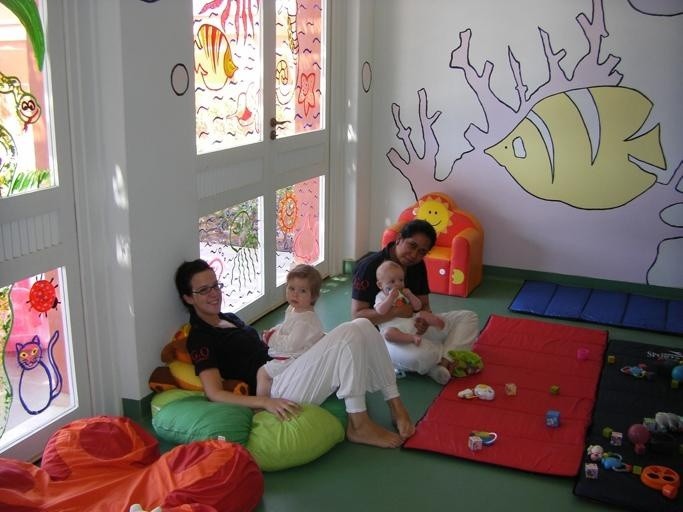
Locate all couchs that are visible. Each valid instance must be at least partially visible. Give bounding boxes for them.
[383,192,485,298]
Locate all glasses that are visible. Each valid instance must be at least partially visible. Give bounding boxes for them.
[191,283,223,295]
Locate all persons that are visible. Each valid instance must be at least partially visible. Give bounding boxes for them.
[372,260,444,346]
[176,261,416,448]
[250,265,323,414]
[352,220,478,386]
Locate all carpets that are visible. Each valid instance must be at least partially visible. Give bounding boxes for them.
[402,309,608,481]
[570,339,683,512]
[508,279,683,340]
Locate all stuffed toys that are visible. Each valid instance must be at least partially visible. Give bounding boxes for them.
[644,412,683,435]
